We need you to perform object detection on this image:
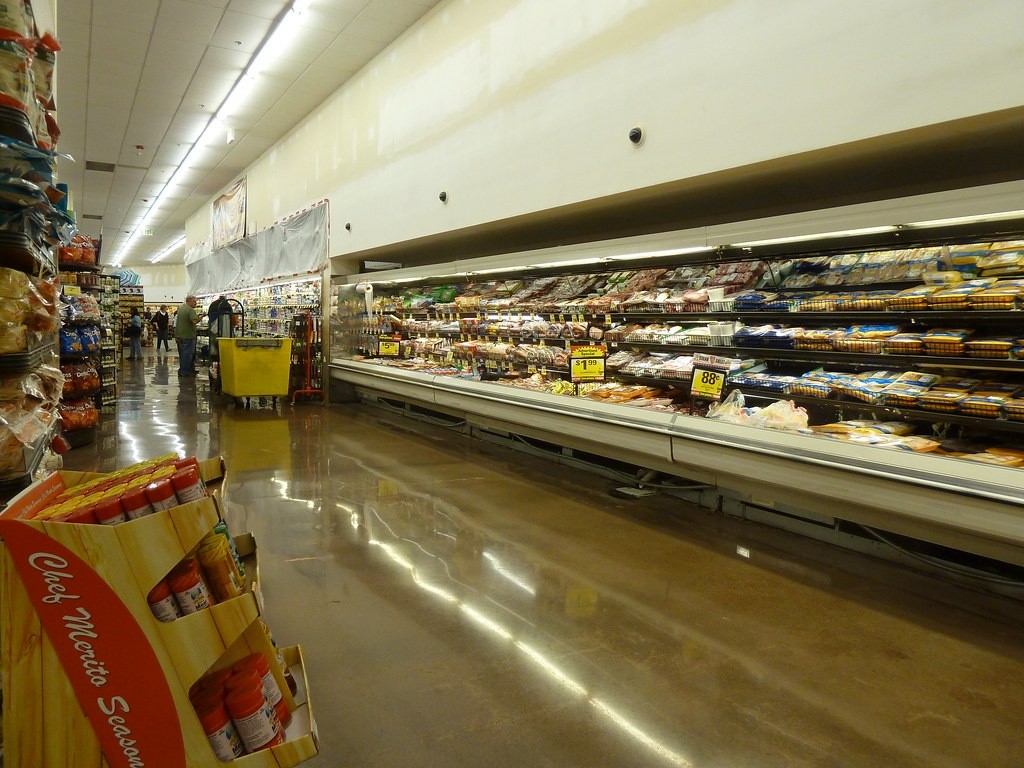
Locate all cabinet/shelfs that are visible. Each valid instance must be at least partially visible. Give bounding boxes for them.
[0,0,1024,766]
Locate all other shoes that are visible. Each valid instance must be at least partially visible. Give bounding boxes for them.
[156,347,172,352]
[179,369,195,376]
[126,357,140,361]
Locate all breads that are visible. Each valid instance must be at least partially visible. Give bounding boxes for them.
[0,1,69,482]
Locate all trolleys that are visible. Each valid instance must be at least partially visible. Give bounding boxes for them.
[214,298,293,414]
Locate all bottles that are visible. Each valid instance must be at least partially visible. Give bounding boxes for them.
[30,460,298,762]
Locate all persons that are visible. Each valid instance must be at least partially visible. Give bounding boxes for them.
[144,307,151,319]
[176,294,206,376]
[207,294,237,367]
[151,304,173,353]
[173,310,178,342]
[125,306,144,362]
